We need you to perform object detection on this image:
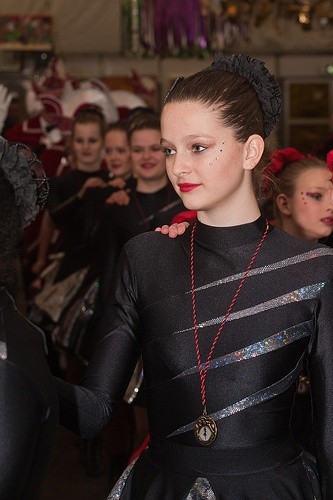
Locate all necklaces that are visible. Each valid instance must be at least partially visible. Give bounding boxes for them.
[191,219,269,447]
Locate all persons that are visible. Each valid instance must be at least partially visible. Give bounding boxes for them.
[0,55,333,500]
